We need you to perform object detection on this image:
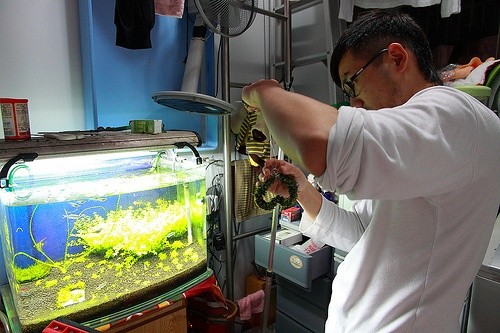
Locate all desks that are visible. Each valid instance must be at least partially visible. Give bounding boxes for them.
[104,297,187,333]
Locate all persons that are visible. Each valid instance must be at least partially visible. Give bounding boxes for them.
[241,11,500,333]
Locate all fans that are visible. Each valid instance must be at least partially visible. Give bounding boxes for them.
[151,0,258,116]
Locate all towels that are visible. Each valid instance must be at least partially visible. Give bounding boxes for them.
[235,290,265,320]
[231,159,273,222]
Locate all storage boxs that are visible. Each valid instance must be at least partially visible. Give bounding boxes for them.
[131,119,163,134]
[247,274,276,325]
[281,207,302,222]
[264,230,302,247]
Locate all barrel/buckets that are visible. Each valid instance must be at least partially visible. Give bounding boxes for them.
[188,297,238,333]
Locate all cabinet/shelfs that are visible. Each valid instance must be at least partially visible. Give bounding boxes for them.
[254,214,470,333]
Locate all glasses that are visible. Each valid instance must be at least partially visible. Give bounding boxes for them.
[342,49,390,104]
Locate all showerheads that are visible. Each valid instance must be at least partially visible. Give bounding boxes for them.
[0,153,38,179]
[174,142,200,157]
